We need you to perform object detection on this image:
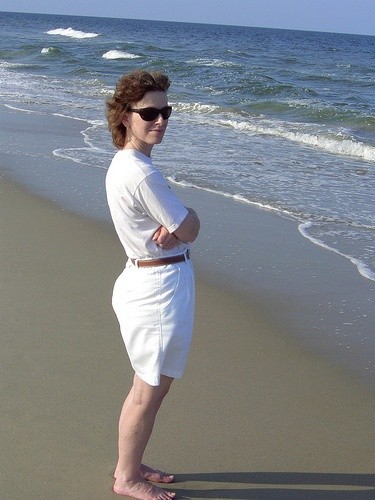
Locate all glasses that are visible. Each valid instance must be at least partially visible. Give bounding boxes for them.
[128,105,172,121]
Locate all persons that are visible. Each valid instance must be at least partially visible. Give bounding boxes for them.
[105,70,201,500]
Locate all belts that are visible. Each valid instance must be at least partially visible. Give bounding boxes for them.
[131,249,191,268]
